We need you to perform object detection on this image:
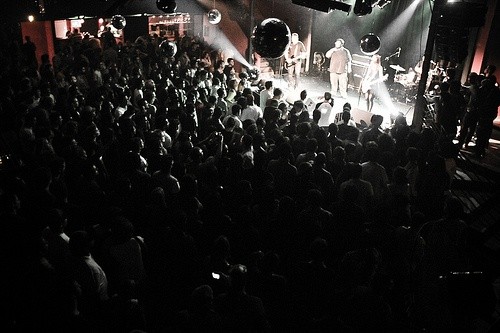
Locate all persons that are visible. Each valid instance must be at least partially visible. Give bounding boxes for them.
[0,1,500,333]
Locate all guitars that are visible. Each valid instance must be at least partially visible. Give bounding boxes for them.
[285,52,306,68]
[362,74,388,93]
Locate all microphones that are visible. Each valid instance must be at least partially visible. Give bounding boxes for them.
[398,47,401,57]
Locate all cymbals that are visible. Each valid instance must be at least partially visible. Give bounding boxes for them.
[390,64,406,71]
